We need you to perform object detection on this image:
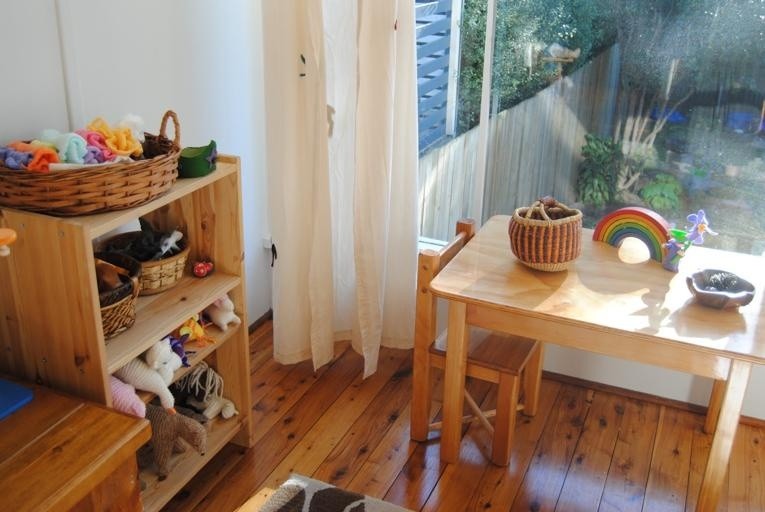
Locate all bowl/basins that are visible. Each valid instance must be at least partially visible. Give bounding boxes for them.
[686,269,758,311]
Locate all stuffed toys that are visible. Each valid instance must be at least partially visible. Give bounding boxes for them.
[661,208,720,274]
[111,292,242,482]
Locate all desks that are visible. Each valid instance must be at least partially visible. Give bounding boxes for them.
[429,214,765,510]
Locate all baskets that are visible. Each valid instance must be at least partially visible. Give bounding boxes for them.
[2,109,182,217]
[507,196,583,273]
[103,231,192,297]
[91,251,143,341]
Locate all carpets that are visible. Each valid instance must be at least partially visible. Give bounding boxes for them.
[260,474,415,512]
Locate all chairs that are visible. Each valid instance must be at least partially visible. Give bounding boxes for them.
[412,220,543,466]
